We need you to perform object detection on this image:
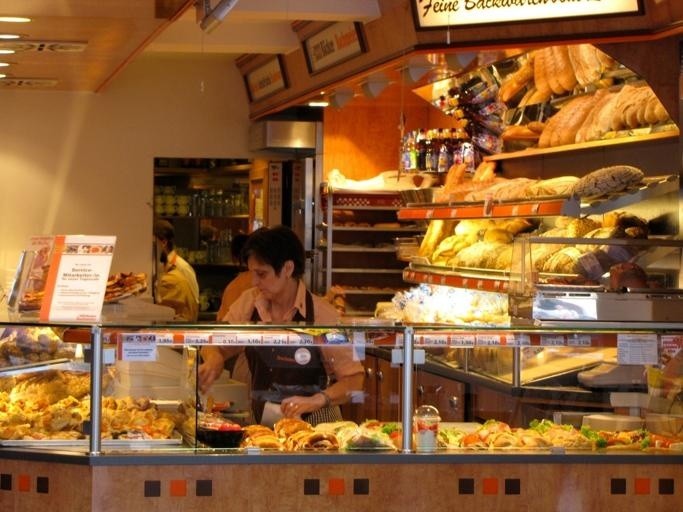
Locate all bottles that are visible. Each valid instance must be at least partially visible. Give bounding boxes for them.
[400,127,475,172]
[206,228,232,265]
[192,190,243,216]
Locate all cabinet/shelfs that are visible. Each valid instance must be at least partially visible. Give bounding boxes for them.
[395,119,683,301]
[1,323,683,512]
[152,157,250,324]
[338,352,630,428]
[319,178,432,316]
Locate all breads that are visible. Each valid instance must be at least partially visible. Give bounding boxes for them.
[374,41,683,325]
[0,327,682,452]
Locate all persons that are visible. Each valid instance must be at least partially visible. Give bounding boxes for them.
[196,225,365,427]
[217,233,256,394]
[152,220,200,324]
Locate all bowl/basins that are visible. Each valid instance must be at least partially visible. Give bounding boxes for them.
[197,428,247,448]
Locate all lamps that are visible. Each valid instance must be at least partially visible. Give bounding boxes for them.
[200,0,238,34]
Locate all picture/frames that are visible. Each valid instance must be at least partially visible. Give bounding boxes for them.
[299,20,366,78]
[240,53,289,105]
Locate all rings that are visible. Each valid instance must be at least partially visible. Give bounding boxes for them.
[290,402,293,407]
[294,405,299,409]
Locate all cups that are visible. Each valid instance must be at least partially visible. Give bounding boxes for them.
[413,405,441,454]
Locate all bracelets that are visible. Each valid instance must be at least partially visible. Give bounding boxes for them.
[315,390,330,409]
